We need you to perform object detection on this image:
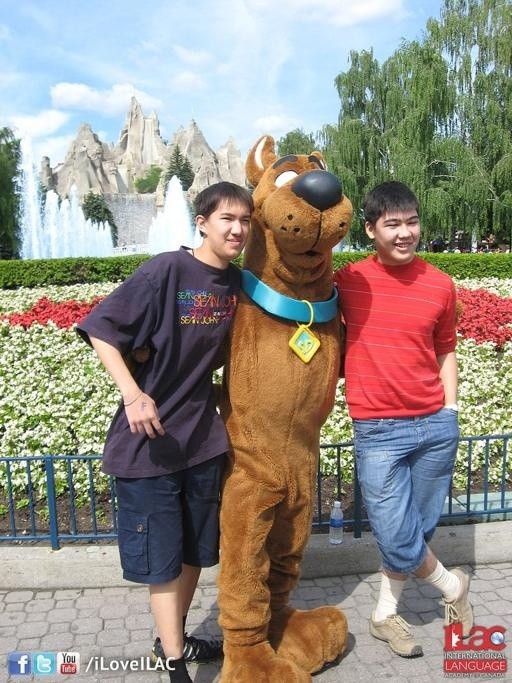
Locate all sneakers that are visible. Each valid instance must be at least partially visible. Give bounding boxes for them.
[437,567,474,640]
[150,632,224,673]
[368,610,422,658]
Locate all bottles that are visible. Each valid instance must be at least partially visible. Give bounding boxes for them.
[328,501,345,545]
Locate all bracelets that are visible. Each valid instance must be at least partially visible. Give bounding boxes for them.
[123,388,142,405]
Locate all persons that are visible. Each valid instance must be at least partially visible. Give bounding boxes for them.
[75,179,259,683]
[203,131,354,683]
[331,179,475,659]
